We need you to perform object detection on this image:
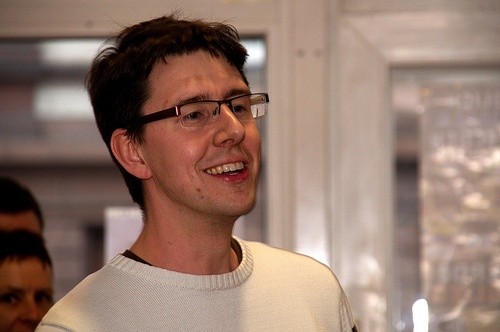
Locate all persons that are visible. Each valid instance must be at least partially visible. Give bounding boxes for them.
[0,228,56,332]
[36,15,360,332]
[0,174,46,239]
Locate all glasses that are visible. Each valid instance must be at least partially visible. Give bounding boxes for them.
[133,93,270,127]
